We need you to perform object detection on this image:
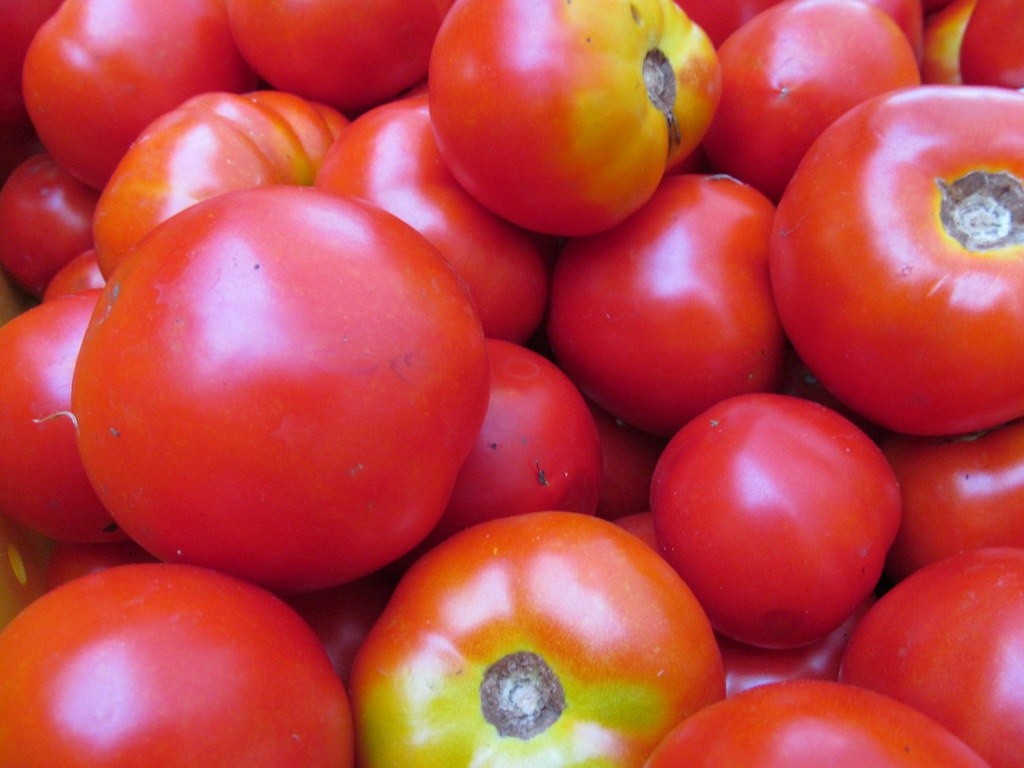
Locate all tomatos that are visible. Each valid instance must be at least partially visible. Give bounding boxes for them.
[1,1,1024,766]
